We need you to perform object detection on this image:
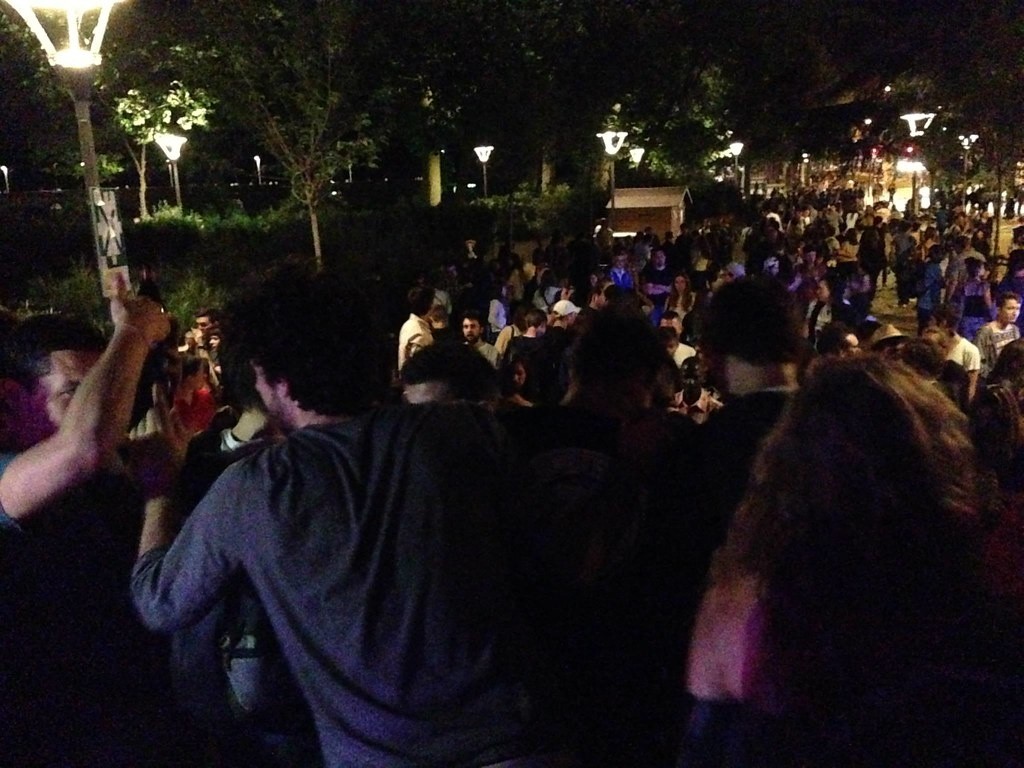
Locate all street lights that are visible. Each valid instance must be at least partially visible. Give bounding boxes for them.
[2,0,116,329]
[900,113,939,215]
[958,134,982,212]
[151,134,186,218]
[473,146,495,199]
[729,141,744,191]
[629,148,646,174]
[595,130,628,232]
[253,156,264,186]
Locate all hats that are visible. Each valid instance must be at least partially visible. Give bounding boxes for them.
[869,324,913,350]
[858,321,880,337]
[817,321,847,355]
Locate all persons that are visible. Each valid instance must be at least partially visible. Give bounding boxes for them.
[0,179,1024,768]
